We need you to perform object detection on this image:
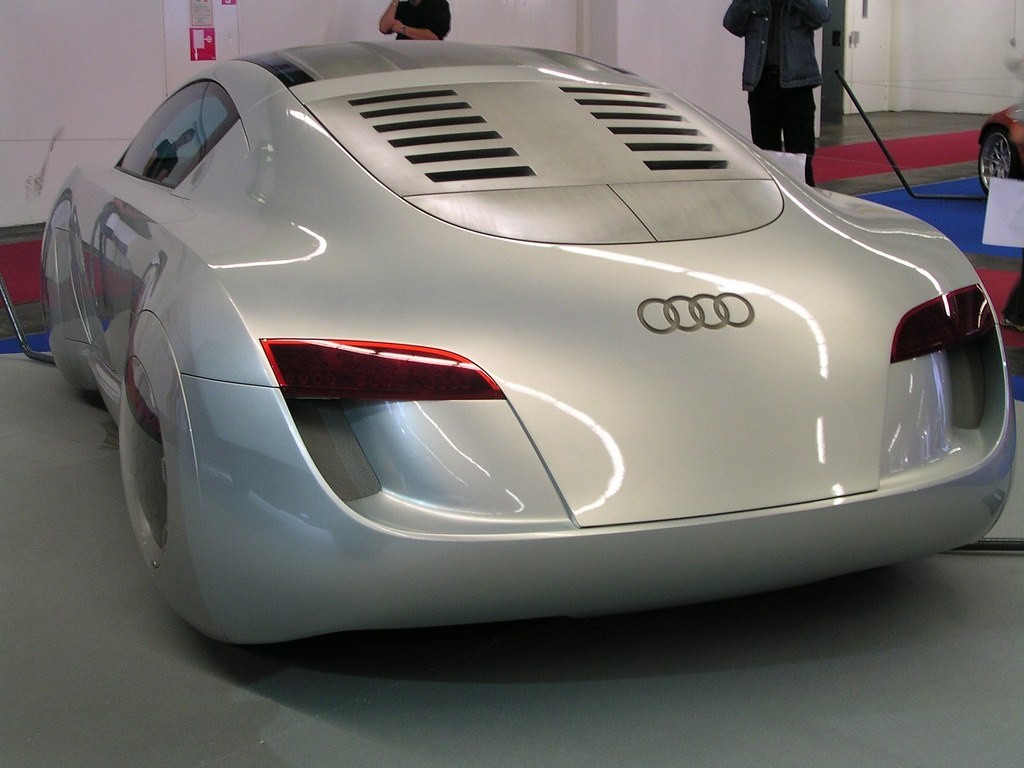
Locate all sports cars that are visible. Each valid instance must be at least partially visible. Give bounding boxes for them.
[37,38,1019,638]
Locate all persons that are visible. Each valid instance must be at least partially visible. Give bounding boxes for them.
[723,0,832,187]
[379,0,451,40]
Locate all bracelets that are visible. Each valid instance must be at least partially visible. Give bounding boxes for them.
[392,1,398,8]
[401,25,406,35]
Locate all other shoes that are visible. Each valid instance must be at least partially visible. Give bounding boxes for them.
[1000,317,1024,332]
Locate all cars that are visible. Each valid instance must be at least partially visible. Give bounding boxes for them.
[977,95,1024,196]
